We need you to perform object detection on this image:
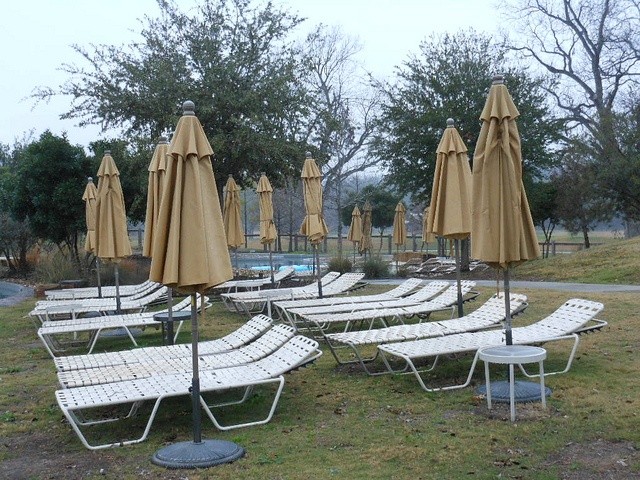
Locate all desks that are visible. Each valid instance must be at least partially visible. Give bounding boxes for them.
[477,345,548,423]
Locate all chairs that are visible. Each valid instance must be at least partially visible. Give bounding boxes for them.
[301,280,477,339]
[55,331,323,452]
[377,298,609,394]
[219,272,340,312]
[41,291,202,326]
[37,295,213,356]
[287,279,449,331]
[52,314,272,370]
[325,292,529,377]
[228,272,364,319]
[45,281,155,296]
[273,278,424,323]
[212,265,296,292]
[28,287,168,331]
[36,283,162,305]
[56,323,297,387]
[44,279,149,291]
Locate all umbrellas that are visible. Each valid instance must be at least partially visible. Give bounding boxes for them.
[422,206,435,254]
[80,175,102,299]
[92,148,143,337]
[299,181,328,276]
[470,74,553,403]
[143,136,173,345]
[301,150,328,301]
[347,203,362,266]
[426,116,475,318]
[255,172,279,288]
[144,96,248,470]
[221,172,246,281]
[391,202,406,272]
[358,200,375,268]
